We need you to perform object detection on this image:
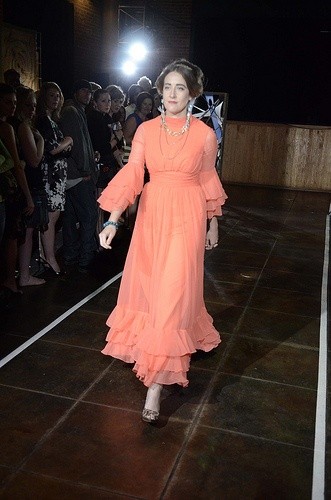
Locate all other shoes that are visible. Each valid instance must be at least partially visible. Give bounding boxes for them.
[142,408,160,423]
[20,276,47,286]
[40,257,61,276]
[64,256,77,264]
[79,252,95,272]
[1,288,24,296]
[0,303,18,311]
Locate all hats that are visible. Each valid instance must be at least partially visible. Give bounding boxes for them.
[106,87,124,98]
[76,79,92,93]
[90,82,101,90]
